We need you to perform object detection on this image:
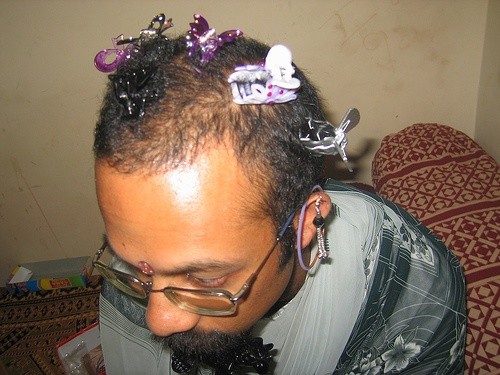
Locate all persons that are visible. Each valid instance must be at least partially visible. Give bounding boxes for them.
[90,29,470,375]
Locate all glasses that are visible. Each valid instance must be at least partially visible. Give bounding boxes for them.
[91,202,300,319]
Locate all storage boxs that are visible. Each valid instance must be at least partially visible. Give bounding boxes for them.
[6,255,94,291]
